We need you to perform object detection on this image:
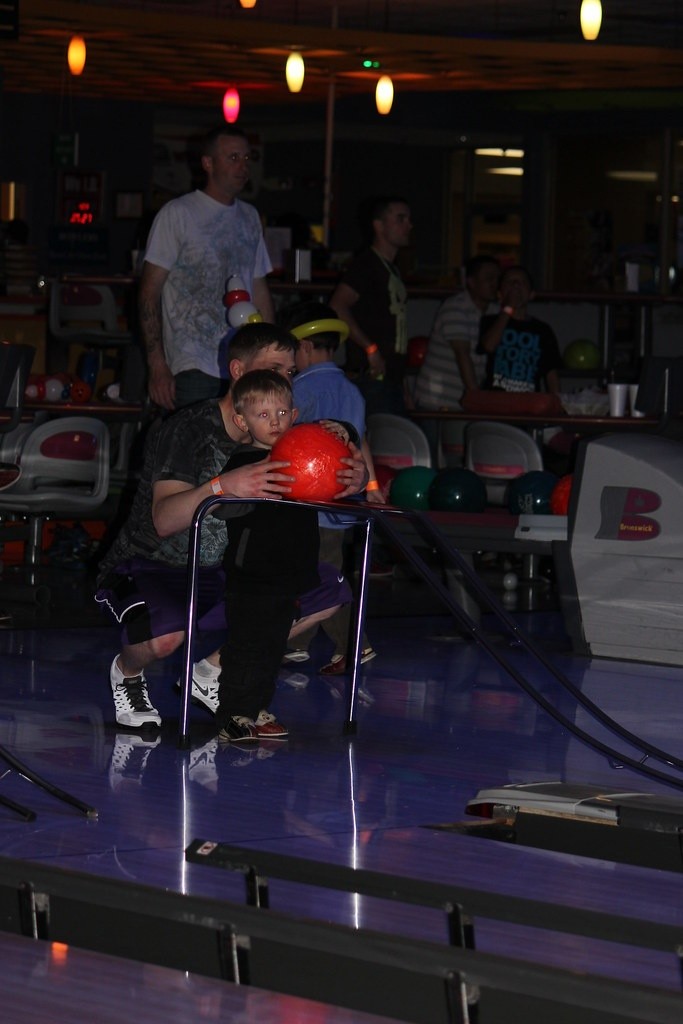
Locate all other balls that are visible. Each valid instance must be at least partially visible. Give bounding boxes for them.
[552,474,571,515]
[271,423,354,503]
[373,465,395,505]
[504,470,555,516]
[430,467,488,513]
[389,465,434,511]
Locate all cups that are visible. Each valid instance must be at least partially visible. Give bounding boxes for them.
[630,384,647,417]
[608,384,627,417]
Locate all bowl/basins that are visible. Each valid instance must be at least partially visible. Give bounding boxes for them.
[554,393,608,416]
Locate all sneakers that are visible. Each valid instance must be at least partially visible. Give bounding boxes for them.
[223,715,259,741]
[108,653,162,730]
[189,734,221,777]
[254,709,290,737]
[176,662,221,713]
[321,675,375,706]
[282,648,310,663]
[109,731,162,781]
[318,648,376,674]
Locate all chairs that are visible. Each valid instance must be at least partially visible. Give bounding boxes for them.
[0,417,111,586]
[366,414,549,627]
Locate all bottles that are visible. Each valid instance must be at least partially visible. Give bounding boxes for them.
[131,238,141,272]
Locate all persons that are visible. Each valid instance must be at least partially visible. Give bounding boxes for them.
[136,125,277,416]
[277,300,387,674]
[555,207,624,291]
[475,265,564,396]
[209,368,362,747]
[328,195,413,417]
[412,254,501,473]
[92,321,370,736]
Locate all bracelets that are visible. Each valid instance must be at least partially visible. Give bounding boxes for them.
[211,475,224,495]
[500,305,516,316]
[366,342,378,356]
